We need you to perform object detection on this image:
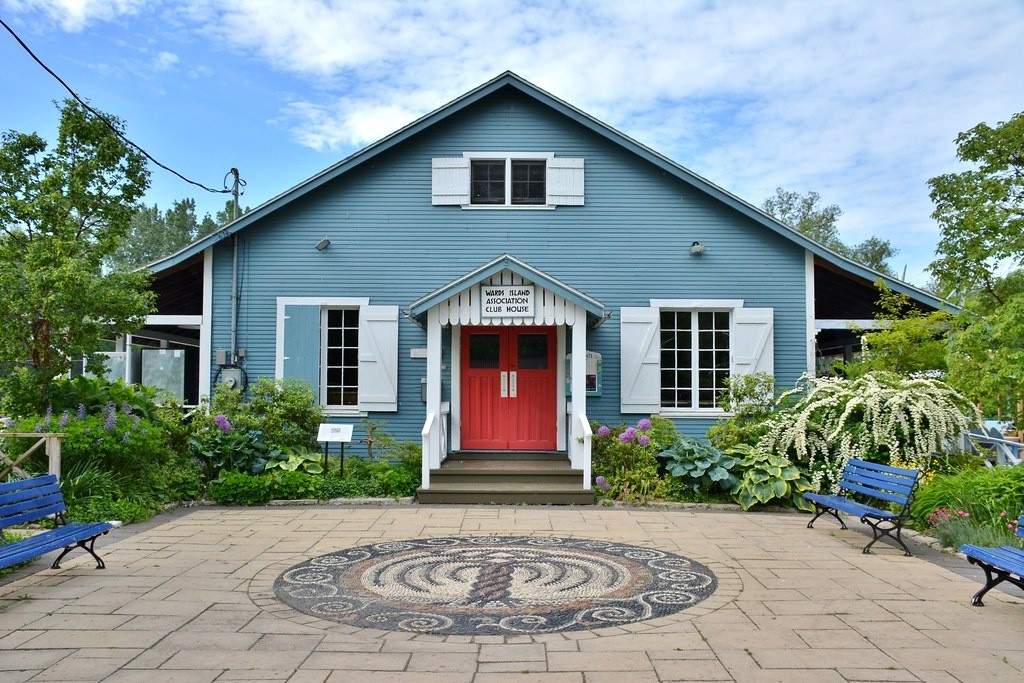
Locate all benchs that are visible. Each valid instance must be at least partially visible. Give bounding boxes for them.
[959,510,1024,606]
[803,458,919,556]
[0,473,111,569]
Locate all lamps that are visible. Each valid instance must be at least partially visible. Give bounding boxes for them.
[690,240,705,253]
